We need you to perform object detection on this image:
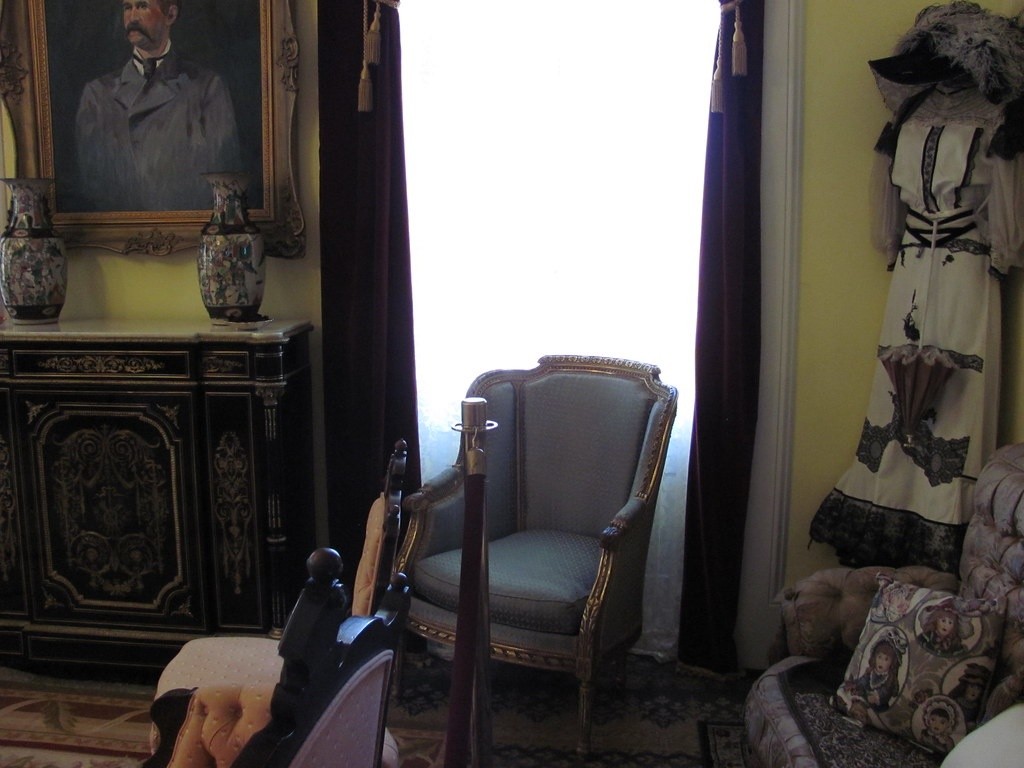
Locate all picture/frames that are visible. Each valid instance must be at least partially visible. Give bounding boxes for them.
[0,0,308,261]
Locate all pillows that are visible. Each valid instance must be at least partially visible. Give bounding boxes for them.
[828,572,1007,759]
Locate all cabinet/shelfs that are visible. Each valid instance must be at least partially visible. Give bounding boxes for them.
[0,319,316,670]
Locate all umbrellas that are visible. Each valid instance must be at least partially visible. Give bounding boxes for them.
[875,218,959,447]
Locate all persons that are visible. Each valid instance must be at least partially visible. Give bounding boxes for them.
[806,0,1024,569]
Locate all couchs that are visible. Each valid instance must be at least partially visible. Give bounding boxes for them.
[742,441,1024,768]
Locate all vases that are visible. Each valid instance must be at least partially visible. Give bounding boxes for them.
[0,178,70,326]
[197,171,269,326]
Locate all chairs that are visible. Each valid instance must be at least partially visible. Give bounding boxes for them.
[141,548,412,768]
[391,355,679,764]
[149,436,407,752]
